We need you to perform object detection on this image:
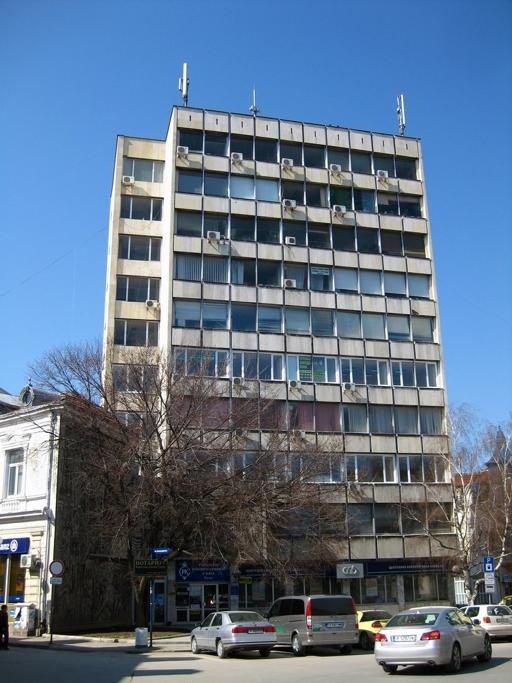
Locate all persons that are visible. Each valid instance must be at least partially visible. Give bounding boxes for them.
[0,605,9,650]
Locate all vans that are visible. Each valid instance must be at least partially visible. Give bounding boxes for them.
[264,594,359,655]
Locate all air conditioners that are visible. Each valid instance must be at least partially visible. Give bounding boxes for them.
[283,278,297,289]
[176,145,189,157]
[281,157,293,167]
[288,380,301,389]
[342,382,356,392]
[231,376,244,387]
[333,204,347,214]
[19,554,36,570]
[285,235,297,246]
[231,151,243,163]
[122,175,135,186]
[146,298,158,309]
[282,198,297,208]
[206,230,221,243]
[376,170,389,179]
[330,163,342,172]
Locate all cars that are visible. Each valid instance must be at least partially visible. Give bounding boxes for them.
[374,606,492,672]
[356,608,393,650]
[190,610,277,659]
[459,604,512,642]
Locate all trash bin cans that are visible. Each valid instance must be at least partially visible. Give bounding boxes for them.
[134,627,148,648]
